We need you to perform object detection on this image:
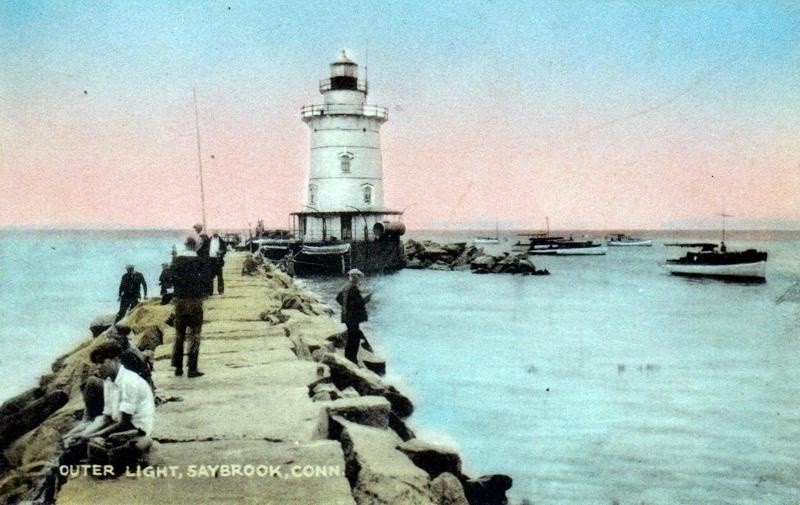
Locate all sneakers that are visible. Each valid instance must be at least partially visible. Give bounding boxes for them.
[175,368,184,377]
[187,370,205,378]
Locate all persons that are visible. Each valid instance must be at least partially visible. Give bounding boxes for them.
[242,246,297,278]
[336,269,369,368]
[61,222,227,481]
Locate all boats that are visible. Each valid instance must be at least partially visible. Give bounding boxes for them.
[607,233,653,247]
[658,248,770,283]
[221,233,243,244]
[473,236,501,245]
[511,236,608,256]
[517,232,551,238]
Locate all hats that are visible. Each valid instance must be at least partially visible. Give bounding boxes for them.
[193,222,204,230]
[349,269,364,275]
